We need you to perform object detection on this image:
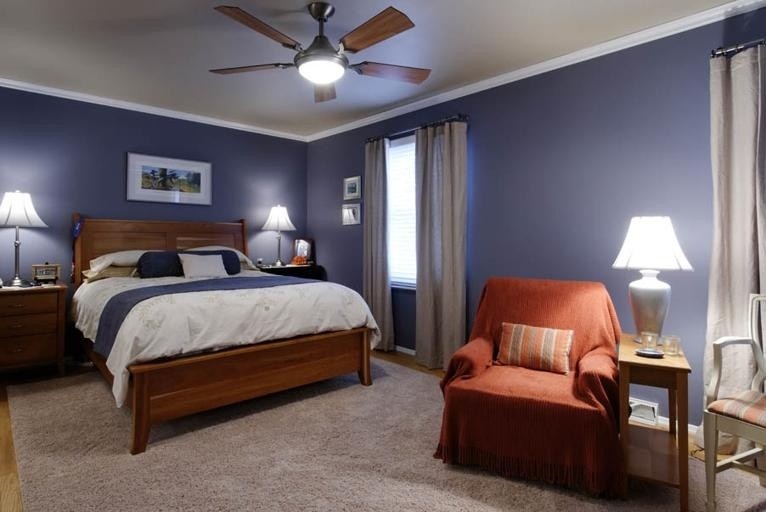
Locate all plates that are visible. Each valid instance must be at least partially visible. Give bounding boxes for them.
[634,349,665,357]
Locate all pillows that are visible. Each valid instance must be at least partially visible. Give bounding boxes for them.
[492,323,574,374]
[178,254,228,278]
[137,250,241,279]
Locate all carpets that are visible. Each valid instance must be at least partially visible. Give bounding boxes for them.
[7,356,766,512]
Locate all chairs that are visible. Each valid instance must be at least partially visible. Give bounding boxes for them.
[703,294,766,512]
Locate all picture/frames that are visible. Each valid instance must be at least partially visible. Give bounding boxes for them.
[126,152,213,205]
[342,203,361,225]
[343,176,361,200]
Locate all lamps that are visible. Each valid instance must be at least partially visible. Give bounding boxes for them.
[209,3,431,103]
[611,216,693,344]
[0,191,48,287]
[261,205,297,266]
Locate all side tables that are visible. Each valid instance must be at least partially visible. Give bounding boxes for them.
[618,334,692,512]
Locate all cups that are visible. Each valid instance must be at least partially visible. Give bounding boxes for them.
[662,336,681,357]
[641,332,658,352]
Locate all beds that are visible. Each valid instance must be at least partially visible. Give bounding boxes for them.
[72,211,376,455]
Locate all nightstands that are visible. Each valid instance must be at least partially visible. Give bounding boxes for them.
[0,283,68,383]
[258,264,313,278]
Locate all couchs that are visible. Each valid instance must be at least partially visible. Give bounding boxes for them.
[434,276,630,498]
